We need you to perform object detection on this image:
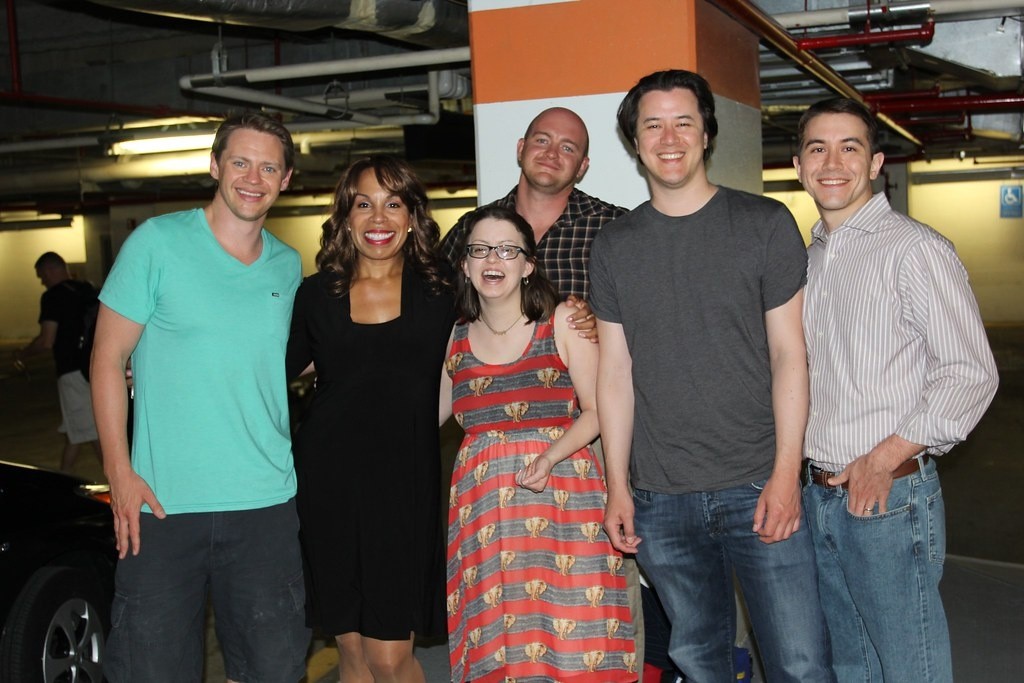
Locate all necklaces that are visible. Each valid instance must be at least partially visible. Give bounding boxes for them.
[479,311,525,334]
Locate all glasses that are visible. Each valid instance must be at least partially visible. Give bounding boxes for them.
[466,244,529,260]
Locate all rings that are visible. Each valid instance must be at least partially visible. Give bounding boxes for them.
[864,507,874,512]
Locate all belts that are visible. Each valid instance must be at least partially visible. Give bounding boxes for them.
[810,453,929,490]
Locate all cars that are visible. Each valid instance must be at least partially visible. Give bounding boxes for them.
[0,460,120,683]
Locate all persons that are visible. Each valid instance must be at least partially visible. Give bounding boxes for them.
[438,207,638,681]
[129,151,599,683]
[12,251,103,468]
[90,113,314,683]
[436,107,685,683]
[791,98,998,681]
[587,70,830,683]
